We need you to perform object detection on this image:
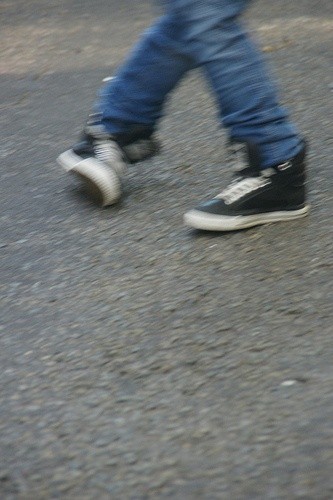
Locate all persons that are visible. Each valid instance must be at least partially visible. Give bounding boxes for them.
[55,1,309,232]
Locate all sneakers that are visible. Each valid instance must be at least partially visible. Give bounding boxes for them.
[57,135,158,206]
[180,141,310,231]
